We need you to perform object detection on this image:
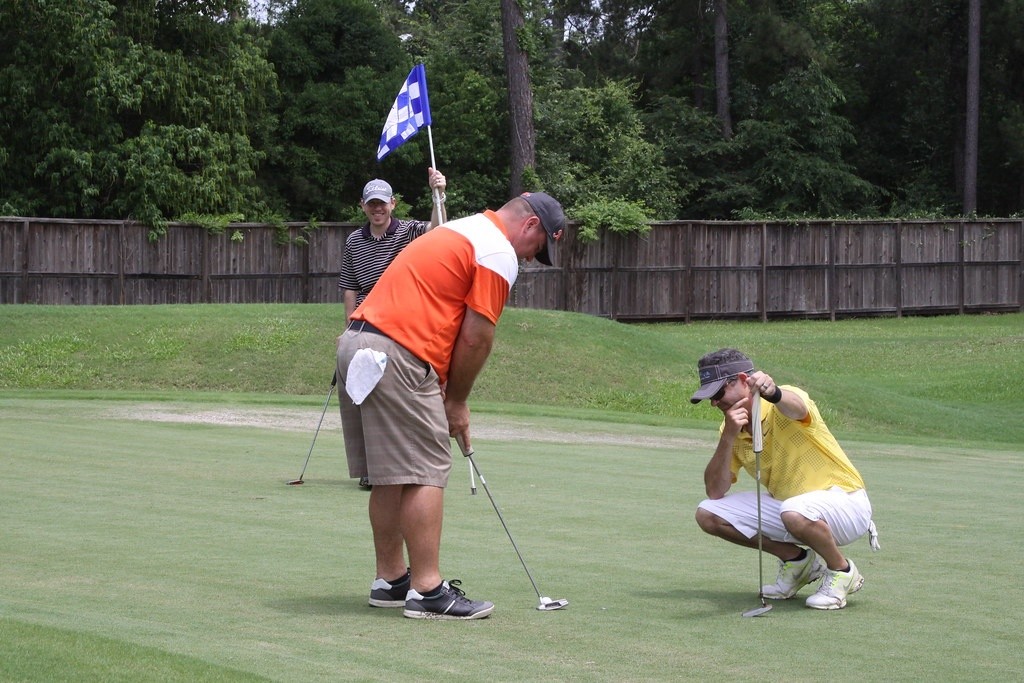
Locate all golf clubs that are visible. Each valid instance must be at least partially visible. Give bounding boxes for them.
[740,389,773,619]
[458,435,570,612]
[283,368,339,487]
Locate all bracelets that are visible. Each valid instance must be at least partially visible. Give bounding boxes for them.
[431,189,447,204]
[760,386,782,403]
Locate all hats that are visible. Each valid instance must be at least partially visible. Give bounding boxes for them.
[519,191,566,269]
[691,359,755,404]
[362,178,393,205]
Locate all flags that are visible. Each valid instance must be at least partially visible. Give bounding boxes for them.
[377,64,432,161]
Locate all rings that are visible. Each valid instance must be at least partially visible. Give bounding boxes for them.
[762,383,768,390]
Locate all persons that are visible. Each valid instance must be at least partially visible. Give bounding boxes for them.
[337,192,566,620]
[690,348,881,609]
[338,167,447,489]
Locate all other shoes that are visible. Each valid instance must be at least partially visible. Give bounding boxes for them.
[359,478,372,490]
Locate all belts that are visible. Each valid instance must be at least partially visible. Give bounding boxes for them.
[347,319,391,338]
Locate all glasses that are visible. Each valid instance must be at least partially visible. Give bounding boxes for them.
[709,379,734,402]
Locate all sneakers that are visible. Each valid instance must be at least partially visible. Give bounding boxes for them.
[368,567,496,621]
[804,558,866,611]
[759,548,827,600]
